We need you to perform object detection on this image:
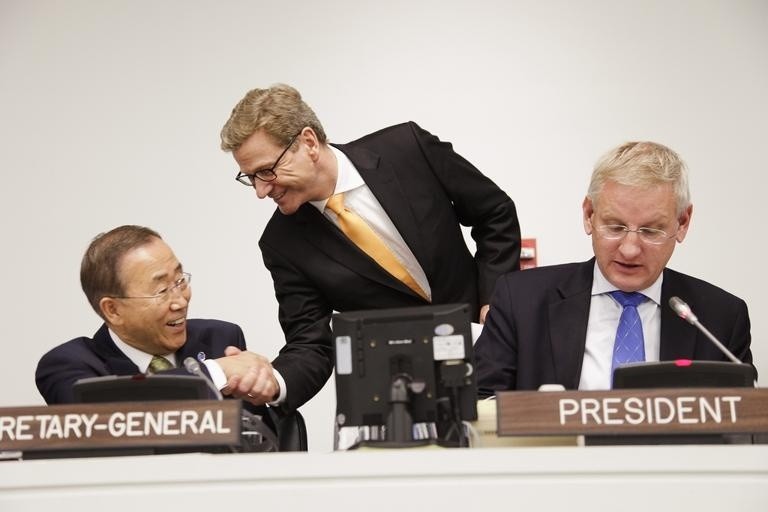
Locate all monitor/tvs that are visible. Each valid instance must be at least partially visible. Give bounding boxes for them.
[332,303,478,449]
[71,375,224,401]
[613,360,754,388]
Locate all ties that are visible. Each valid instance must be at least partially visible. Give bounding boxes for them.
[324,195,432,303]
[148,355,175,374]
[611,289,651,388]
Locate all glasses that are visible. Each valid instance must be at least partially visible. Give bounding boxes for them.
[235,136,303,187]
[588,216,679,245]
[109,271,190,305]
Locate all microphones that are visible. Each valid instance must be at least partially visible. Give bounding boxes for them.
[668,296,741,363]
[184,357,224,400]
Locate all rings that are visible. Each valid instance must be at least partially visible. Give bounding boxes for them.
[246,393,258,399]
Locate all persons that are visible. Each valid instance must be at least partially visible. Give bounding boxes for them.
[35,225,276,453]
[467,141,751,398]
[217,80,521,415]
